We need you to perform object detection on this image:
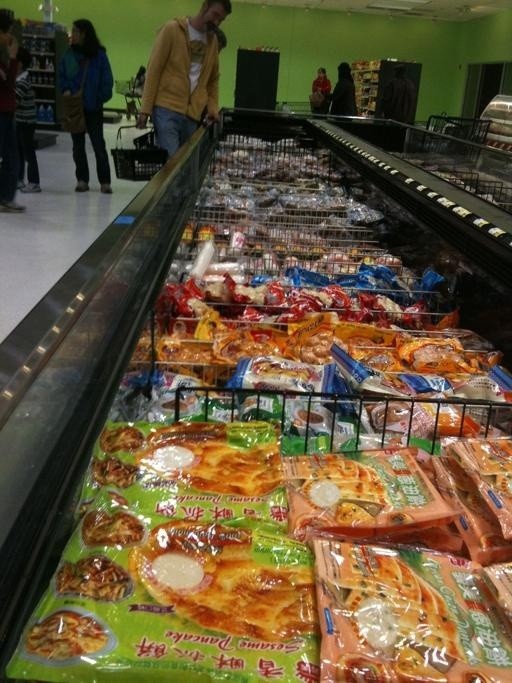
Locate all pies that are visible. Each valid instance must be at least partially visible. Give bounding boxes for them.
[279,447,461,538]
[399,438,512,642]
[73,420,290,529]
[4,487,321,683]
[308,539,512,683]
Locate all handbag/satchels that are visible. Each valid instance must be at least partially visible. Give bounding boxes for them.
[307,87,327,108]
[53,56,93,132]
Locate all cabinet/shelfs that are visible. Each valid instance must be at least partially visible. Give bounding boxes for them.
[351,60,422,126]
[234,49,280,111]
[19,29,68,131]
[476,94,512,151]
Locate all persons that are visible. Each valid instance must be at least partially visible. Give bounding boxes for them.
[196,28,227,130]
[132,63,146,97]
[329,62,358,120]
[0,12,26,214]
[11,46,43,194]
[379,61,418,129]
[312,65,332,96]
[135,0,232,220]
[55,18,113,194]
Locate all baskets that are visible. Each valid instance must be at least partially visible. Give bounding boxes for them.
[110,125,169,182]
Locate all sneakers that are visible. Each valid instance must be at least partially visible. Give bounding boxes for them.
[0,202,27,213]
[16,181,42,193]
[74,181,90,193]
[100,184,113,194]
